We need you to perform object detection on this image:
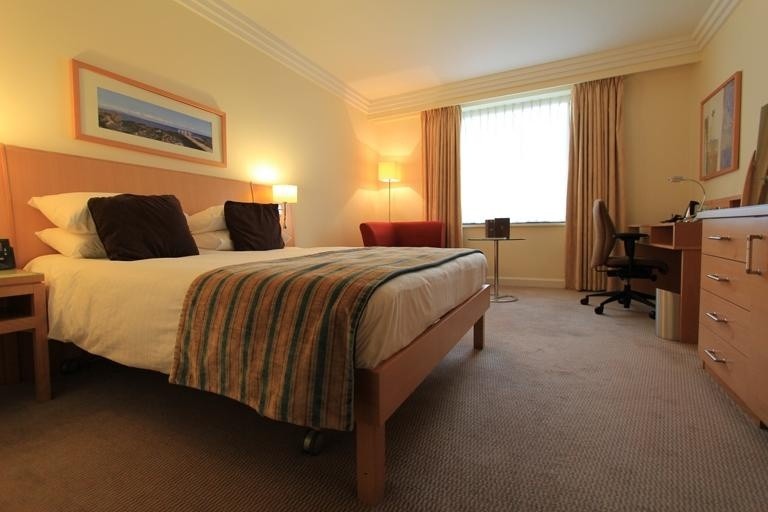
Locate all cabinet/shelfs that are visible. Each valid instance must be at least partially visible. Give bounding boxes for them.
[696,204,768,430]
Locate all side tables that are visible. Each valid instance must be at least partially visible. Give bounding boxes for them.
[468,237,527,302]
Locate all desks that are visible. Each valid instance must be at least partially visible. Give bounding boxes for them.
[627,195,742,345]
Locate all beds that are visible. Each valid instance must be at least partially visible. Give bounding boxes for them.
[0,140,490,504]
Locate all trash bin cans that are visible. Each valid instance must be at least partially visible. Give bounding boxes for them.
[655,288,681,340]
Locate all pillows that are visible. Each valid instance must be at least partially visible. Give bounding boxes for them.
[26,193,286,260]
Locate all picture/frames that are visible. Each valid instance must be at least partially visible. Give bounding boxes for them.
[70,57,227,168]
[699,71,743,181]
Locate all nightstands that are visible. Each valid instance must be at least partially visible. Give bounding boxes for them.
[0,268,52,403]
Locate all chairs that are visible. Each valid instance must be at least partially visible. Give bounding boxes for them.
[579,199,667,318]
[360,221,446,248]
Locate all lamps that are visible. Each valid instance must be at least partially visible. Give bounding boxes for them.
[273,183,298,229]
[378,160,401,222]
